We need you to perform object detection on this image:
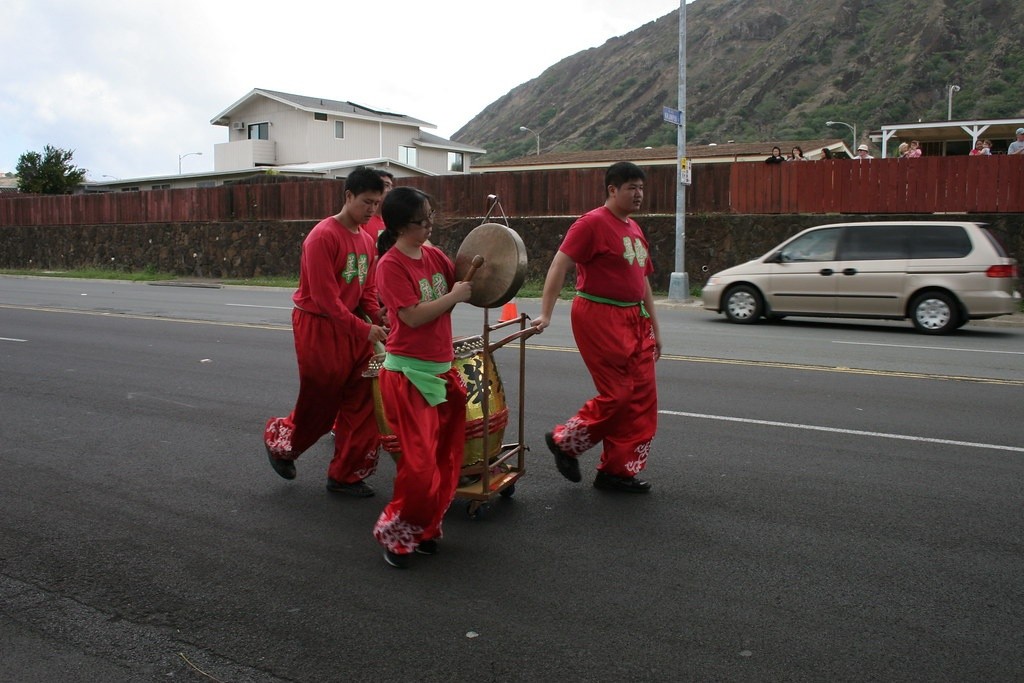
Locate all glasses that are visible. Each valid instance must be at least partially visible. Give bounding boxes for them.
[384,183,396,188]
[412,208,437,228]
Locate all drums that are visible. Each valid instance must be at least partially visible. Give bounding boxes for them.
[362,335,508,487]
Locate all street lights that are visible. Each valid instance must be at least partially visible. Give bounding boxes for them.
[826,122,857,156]
[178,152,202,175]
[519,126,539,156]
[948,85,961,121]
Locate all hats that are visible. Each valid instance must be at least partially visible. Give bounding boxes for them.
[1016,128,1024,135]
[857,144,869,154]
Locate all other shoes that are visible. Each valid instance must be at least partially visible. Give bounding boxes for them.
[326,475,377,497]
[266,447,297,480]
[414,539,437,554]
[383,550,410,568]
[544,430,582,483]
[593,472,651,494]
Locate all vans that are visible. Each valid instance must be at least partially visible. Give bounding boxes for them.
[700,222,1021,336]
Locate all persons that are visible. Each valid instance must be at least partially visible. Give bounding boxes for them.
[327,171,473,568]
[969,139,992,155]
[786,146,807,161]
[262,166,388,499]
[898,140,922,158]
[765,146,784,164]
[853,144,874,159]
[531,162,663,492]
[819,148,831,160]
[1007,128,1024,155]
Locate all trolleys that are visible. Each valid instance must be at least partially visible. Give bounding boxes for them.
[394,313,544,523]
